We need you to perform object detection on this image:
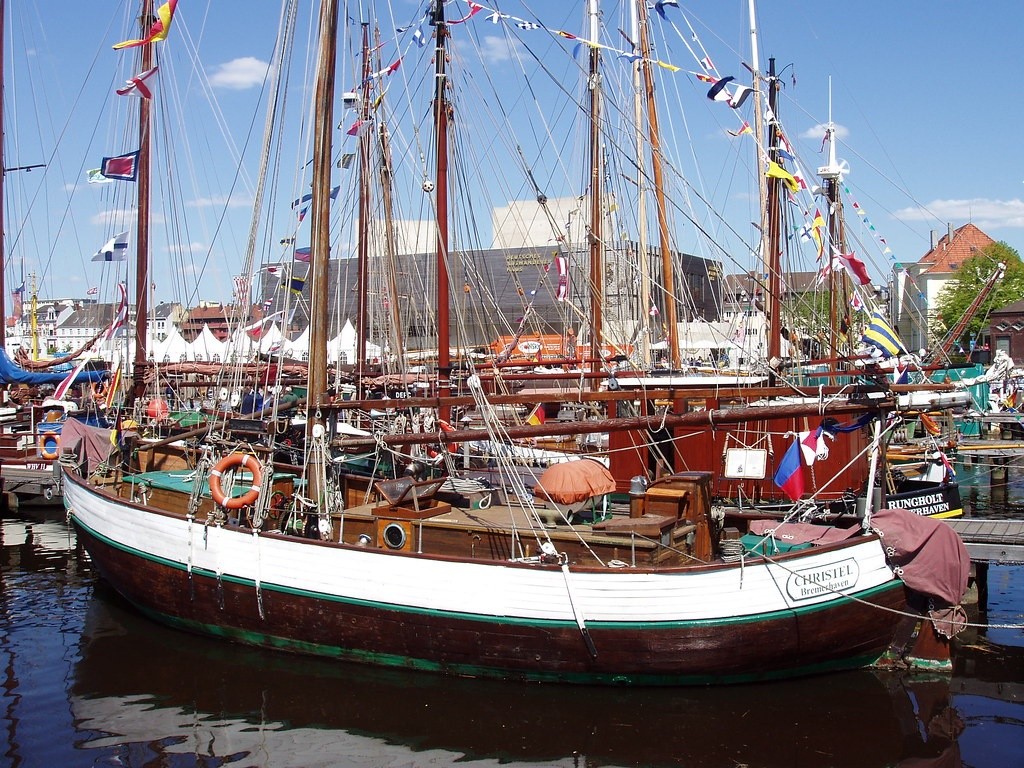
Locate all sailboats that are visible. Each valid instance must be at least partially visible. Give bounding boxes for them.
[0,0,1004,689]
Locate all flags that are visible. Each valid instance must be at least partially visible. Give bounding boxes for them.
[839,251,872,286]
[91,231,128,261]
[112,0,178,50]
[51,283,127,400]
[526,402,547,426]
[295,245,331,264]
[233,277,250,302]
[292,184,340,221]
[774,437,809,502]
[861,312,901,358]
[85,149,140,185]
[939,453,958,489]
[765,160,799,195]
[798,432,831,466]
[249,1,436,343]
[447,0,980,385]
[849,290,862,311]
[555,253,570,301]
[1006,387,1018,407]
[116,66,159,99]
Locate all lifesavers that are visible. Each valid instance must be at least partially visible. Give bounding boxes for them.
[39,430,61,460]
[419,419,459,458]
[209,454,265,509]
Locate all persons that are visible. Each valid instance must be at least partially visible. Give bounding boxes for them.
[240,383,299,418]
[381,390,391,404]
[919,346,927,360]
[165,379,177,406]
